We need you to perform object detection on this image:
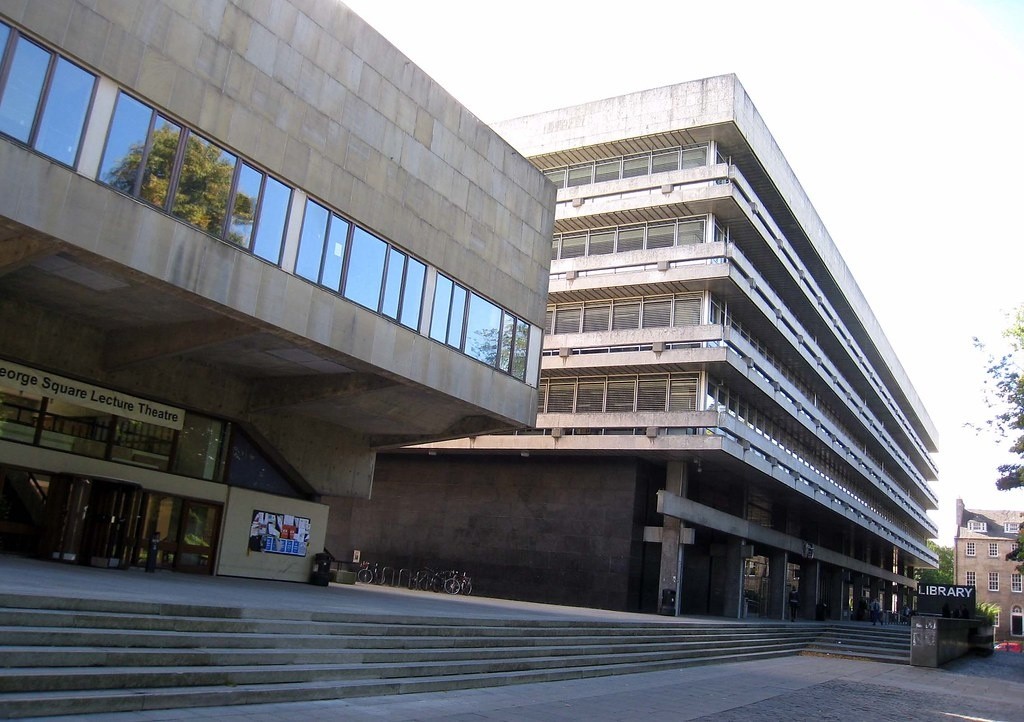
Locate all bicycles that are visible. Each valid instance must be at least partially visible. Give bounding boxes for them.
[358,560,385,584]
[412,566,473,595]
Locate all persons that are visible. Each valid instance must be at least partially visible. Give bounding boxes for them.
[789,585,800,622]
[856,596,915,626]
[145,532,161,574]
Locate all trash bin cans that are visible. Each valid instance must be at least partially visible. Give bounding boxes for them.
[815,602,827,622]
[309,552,333,587]
[658,587,680,617]
